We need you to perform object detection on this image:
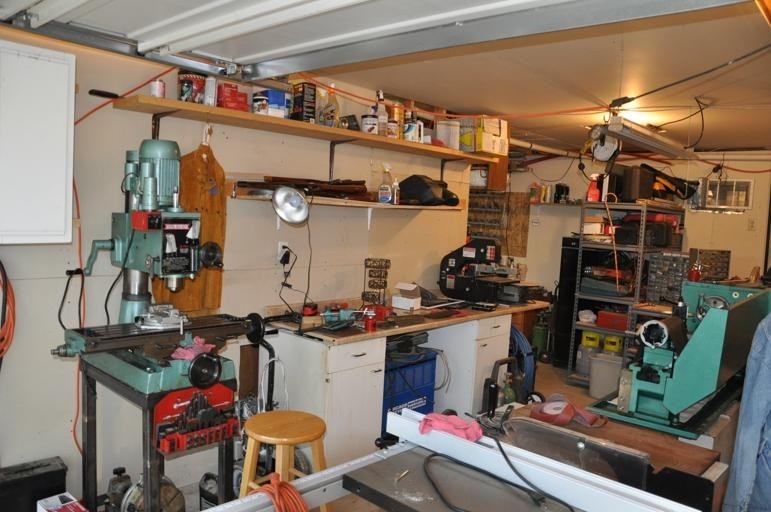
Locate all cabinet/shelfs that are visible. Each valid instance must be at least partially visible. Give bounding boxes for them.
[417,312,512,422]
[109,85,502,232]
[566,196,687,388]
[258,322,387,470]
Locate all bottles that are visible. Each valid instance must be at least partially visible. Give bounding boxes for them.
[254,96,268,114]
[362,115,378,135]
[388,120,398,138]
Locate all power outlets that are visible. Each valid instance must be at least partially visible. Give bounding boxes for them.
[277,241,288,260]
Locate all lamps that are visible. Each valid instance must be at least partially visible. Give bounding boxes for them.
[270,183,326,335]
[608,111,684,161]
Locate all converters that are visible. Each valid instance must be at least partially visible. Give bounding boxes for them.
[280,251,290,264]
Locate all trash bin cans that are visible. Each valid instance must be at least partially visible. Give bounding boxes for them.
[587,353,632,399]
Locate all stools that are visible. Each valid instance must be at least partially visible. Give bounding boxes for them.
[237,410,332,512]
